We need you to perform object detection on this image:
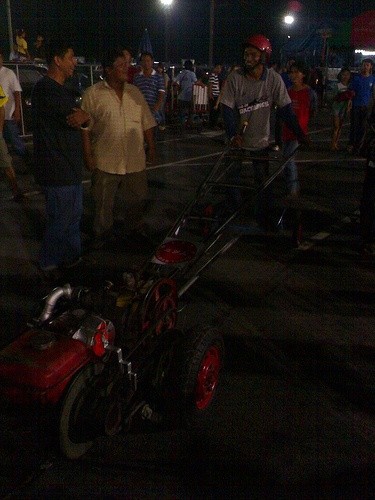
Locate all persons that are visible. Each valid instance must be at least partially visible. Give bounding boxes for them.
[0,28,375,283]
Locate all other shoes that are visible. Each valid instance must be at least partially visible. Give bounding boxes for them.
[77,256,95,267]
[347,145,354,154]
[331,145,340,152]
[14,193,29,201]
[281,193,298,202]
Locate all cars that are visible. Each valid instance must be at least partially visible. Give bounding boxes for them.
[2,60,104,132]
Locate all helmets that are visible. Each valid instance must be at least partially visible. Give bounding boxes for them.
[245,34,273,55]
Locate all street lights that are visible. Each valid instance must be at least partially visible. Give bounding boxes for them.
[160,0,174,65]
